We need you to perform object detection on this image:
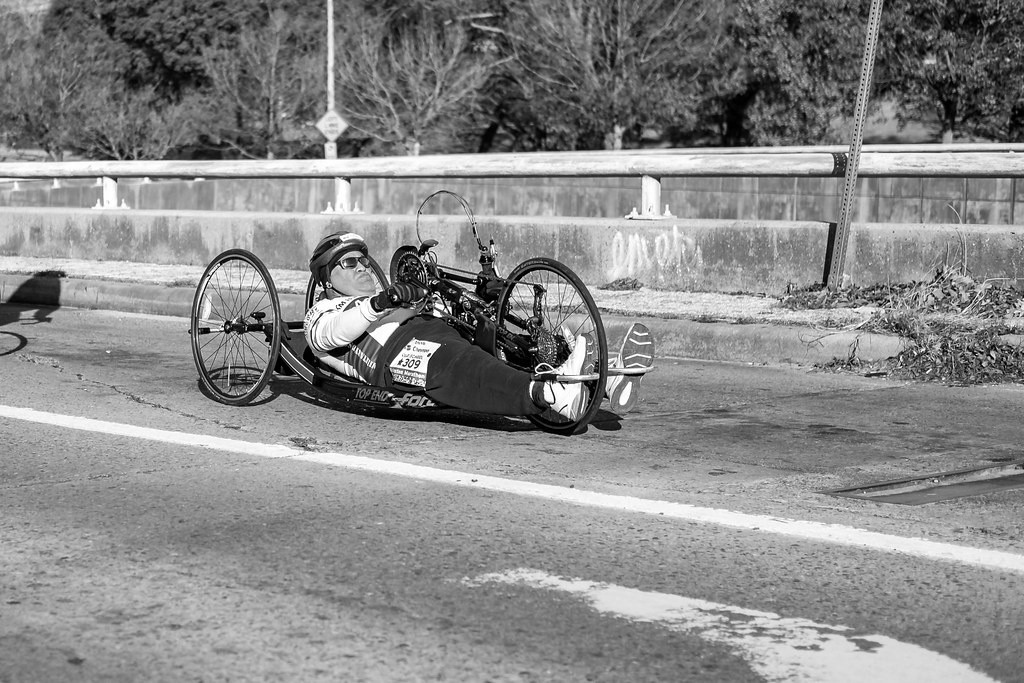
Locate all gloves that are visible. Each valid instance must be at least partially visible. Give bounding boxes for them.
[371,283,418,312]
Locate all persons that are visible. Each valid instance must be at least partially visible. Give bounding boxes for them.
[303,231,655,423]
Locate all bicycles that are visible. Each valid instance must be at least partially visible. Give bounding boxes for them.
[187,187,656,436]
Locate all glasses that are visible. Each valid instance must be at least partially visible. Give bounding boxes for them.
[335,256,370,269]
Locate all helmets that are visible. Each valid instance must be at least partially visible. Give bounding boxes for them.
[310,231,368,283]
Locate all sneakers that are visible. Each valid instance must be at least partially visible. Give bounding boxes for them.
[533,333,596,422]
[605,324,655,415]
